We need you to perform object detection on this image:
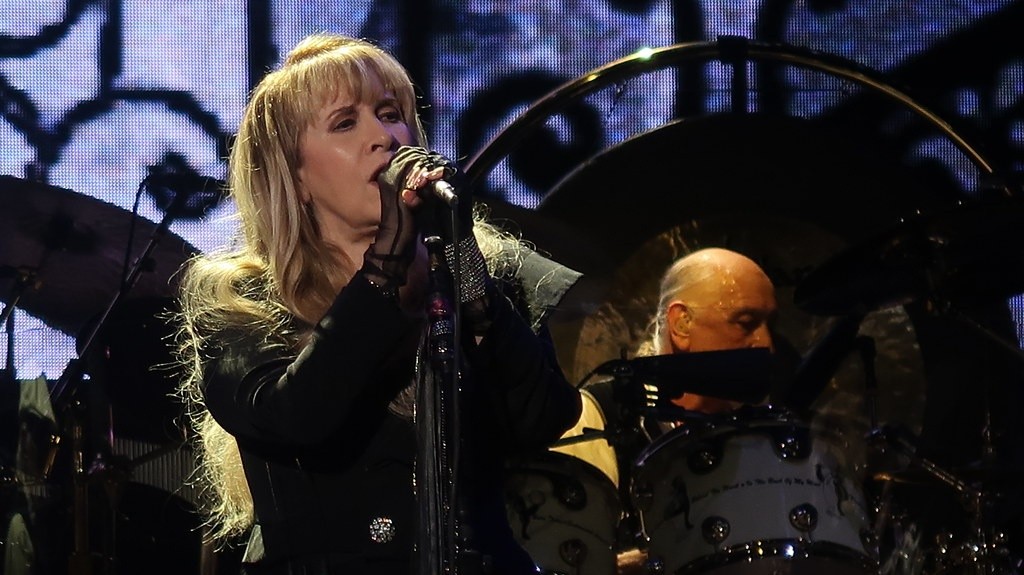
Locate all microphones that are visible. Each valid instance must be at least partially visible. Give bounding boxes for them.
[426,178,458,210]
[149,173,230,197]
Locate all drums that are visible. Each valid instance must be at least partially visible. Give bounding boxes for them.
[621,404,879,575]
[506,447,628,575]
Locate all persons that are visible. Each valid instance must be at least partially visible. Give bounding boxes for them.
[176,30,591,575]
[545,245,779,575]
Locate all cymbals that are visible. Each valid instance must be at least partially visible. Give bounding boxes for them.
[0,173,204,345]
